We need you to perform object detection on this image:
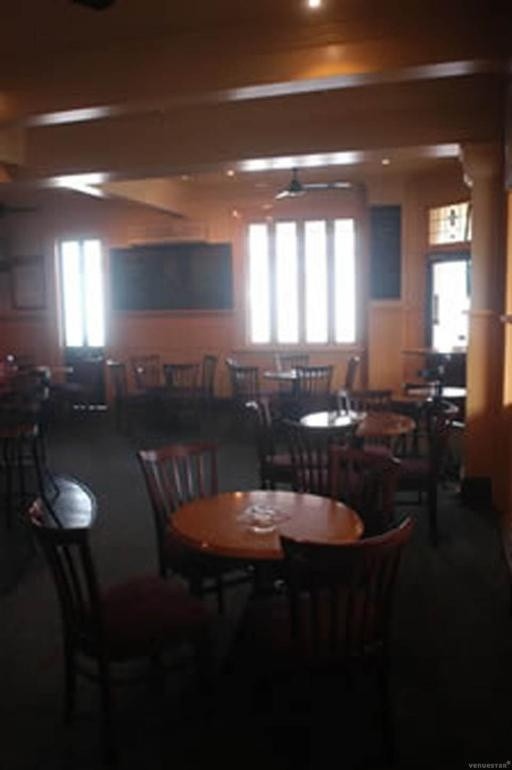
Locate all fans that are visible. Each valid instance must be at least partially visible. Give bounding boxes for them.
[251,166,352,211]
